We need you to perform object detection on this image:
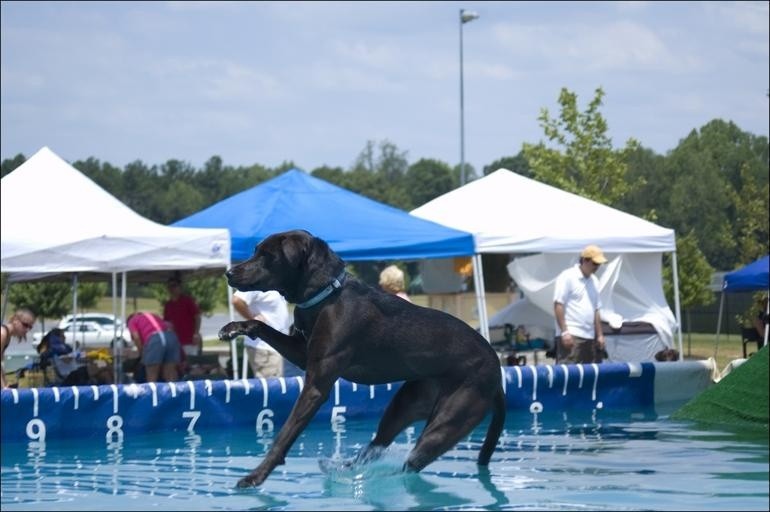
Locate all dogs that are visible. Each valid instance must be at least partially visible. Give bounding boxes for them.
[218,231,504,490]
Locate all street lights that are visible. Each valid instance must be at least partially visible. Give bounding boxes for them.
[459,8,480,185]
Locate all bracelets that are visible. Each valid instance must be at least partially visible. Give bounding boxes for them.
[561,329,569,336]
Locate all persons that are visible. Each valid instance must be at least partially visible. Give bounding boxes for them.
[755,296,770,350]
[552,244,608,365]
[162,278,204,380]
[45,329,87,381]
[379,265,413,304]
[0,307,35,390]
[125,311,180,383]
[230,288,292,377]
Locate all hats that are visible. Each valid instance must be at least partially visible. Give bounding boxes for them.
[580,245,608,265]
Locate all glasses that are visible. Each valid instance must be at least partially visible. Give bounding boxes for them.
[16,317,33,329]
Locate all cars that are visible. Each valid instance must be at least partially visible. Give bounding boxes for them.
[32,313,136,348]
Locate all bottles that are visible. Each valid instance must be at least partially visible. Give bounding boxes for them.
[517,325,525,345]
